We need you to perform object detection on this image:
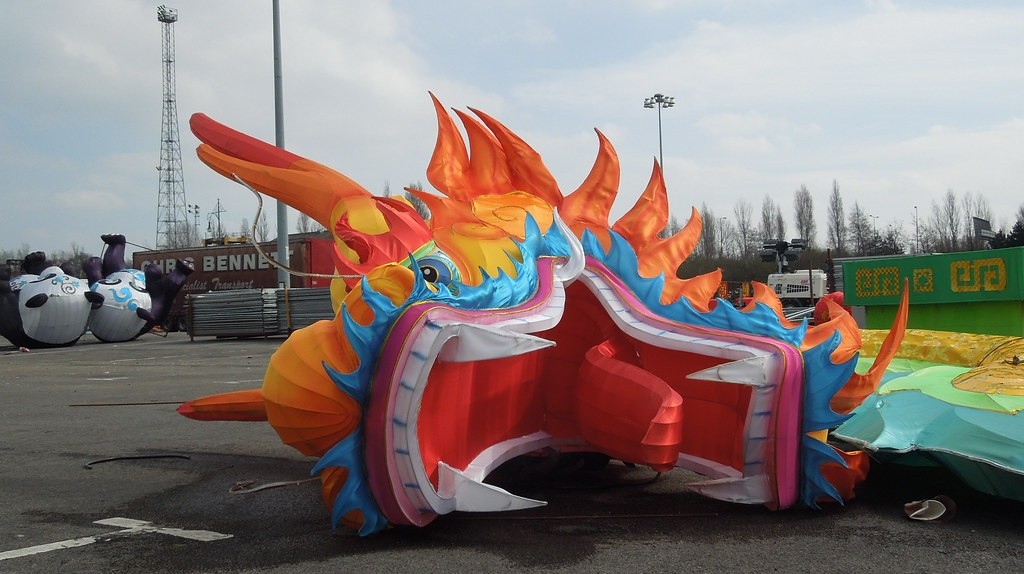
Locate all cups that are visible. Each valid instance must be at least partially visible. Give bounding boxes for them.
[905,500,946,520]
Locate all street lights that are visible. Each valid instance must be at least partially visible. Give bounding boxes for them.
[913,205,918,254]
[720,217,726,256]
[644,91,676,240]
[870,214,880,255]
